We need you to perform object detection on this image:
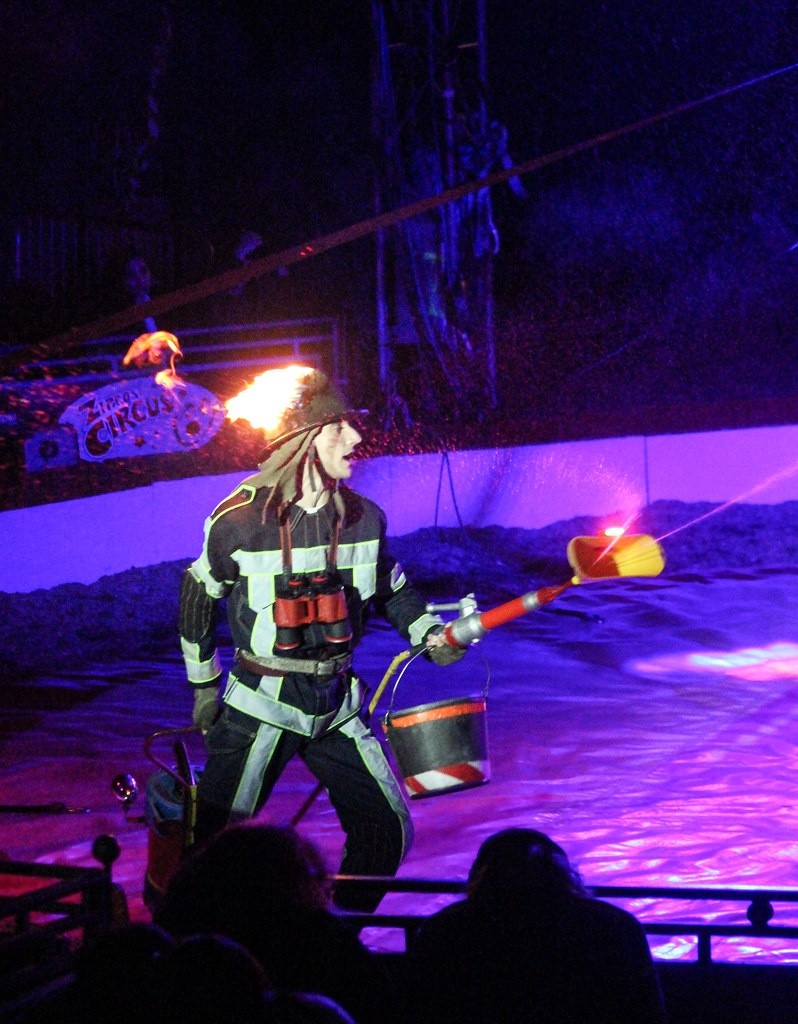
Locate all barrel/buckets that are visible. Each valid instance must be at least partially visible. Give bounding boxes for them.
[378,644,494,800]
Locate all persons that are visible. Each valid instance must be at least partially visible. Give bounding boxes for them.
[99,251,196,335]
[98,820,671,1023]
[176,367,468,917]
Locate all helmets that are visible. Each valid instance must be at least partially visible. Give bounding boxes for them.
[257,368,370,459]
